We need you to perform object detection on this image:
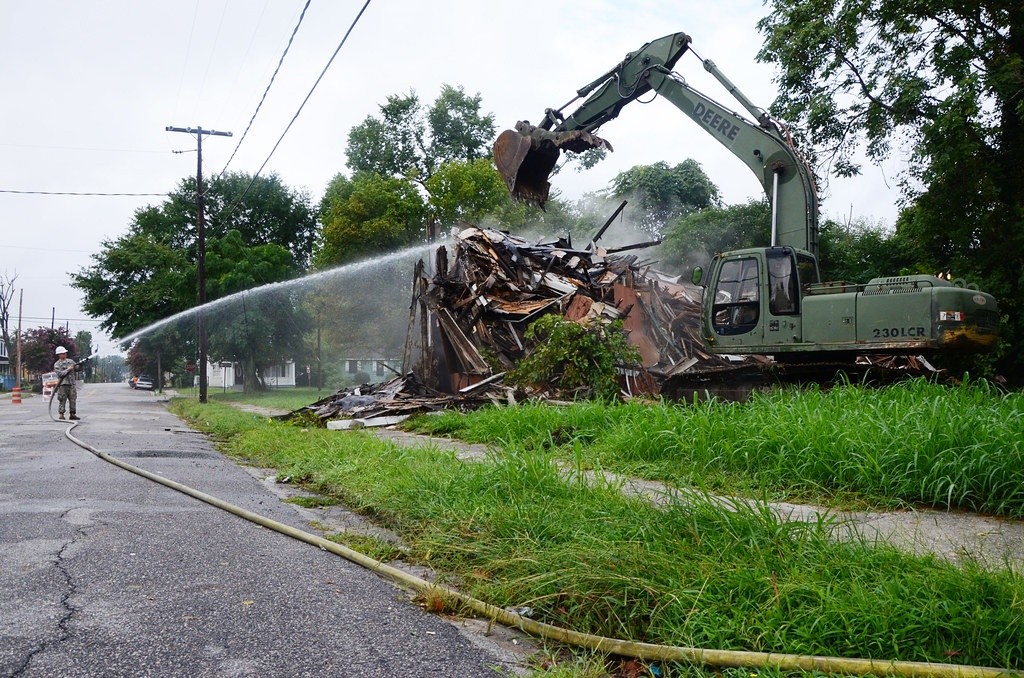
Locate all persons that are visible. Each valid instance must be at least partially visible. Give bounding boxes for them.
[133,375,138,389]
[54,345,84,420]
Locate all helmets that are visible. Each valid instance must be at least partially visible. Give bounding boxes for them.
[55,346,69,354]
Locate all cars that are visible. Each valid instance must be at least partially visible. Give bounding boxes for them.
[129,374,154,390]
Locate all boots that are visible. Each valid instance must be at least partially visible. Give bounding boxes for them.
[69,412,80,420]
[59,413,65,419]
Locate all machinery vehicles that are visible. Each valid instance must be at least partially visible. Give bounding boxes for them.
[494,35,1004,383]
